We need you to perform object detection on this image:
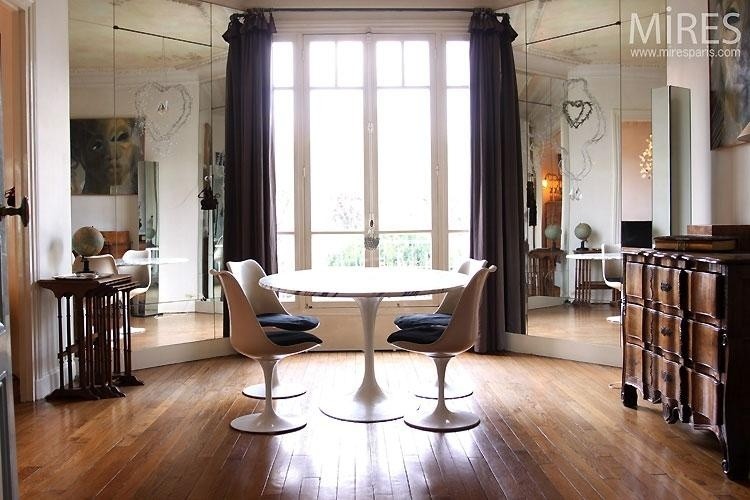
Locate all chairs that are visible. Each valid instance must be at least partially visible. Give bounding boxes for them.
[110,249,151,334]
[211,257,498,434]
[601,243,624,322]
[73,254,124,341]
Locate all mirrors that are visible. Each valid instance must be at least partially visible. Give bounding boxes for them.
[491,1,667,347]
[516,71,562,297]
[67,1,249,357]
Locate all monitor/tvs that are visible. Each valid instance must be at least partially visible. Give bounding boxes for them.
[621,221,652,253]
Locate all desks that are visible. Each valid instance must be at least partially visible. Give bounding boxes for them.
[113,258,188,267]
[528,248,561,297]
[38,272,144,400]
[566,252,622,261]
[574,249,623,308]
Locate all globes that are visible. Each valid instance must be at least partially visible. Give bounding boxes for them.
[544,223,563,251]
[72,226,105,276]
[575,223,592,251]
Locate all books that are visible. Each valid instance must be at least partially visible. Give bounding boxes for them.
[653,234,738,254]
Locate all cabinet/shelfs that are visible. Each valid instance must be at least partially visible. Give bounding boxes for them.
[620,246,750,481]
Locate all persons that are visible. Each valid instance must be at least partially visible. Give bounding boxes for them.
[71,118,138,195]
